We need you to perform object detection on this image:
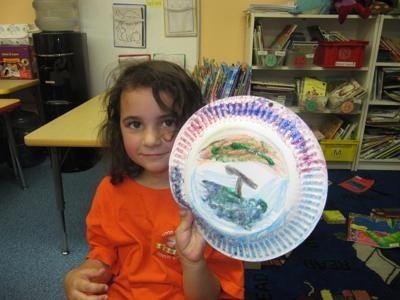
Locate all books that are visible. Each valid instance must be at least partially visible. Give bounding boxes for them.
[383,84,400,102]
[192,56,253,105]
[295,76,327,107]
[365,108,400,134]
[337,175,375,193]
[278,39,291,67]
[322,210,347,224]
[288,40,319,52]
[372,70,384,100]
[379,35,400,63]
[359,134,400,160]
[243,3,288,15]
[370,207,400,223]
[383,67,400,85]
[253,19,264,65]
[329,30,349,42]
[251,77,297,93]
[268,22,298,52]
[289,32,306,41]
[307,25,324,40]
[327,77,368,110]
[312,116,360,141]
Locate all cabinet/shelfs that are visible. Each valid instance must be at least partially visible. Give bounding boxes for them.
[354,15,400,171]
[243,11,380,172]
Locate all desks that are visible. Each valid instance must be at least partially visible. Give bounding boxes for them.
[0,79,41,180]
[0,99,29,191]
[24,87,115,255]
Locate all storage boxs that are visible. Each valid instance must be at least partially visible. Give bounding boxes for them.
[256,48,287,67]
[296,95,328,112]
[313,40,369,69]
[320,139,360,162]
[285,52,315,66]
[328,96,362,115]
[255,91,293,115]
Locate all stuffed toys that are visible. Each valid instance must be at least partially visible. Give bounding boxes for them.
[332,0,371,24]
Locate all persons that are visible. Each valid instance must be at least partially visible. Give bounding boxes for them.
[64,55,246,300]
